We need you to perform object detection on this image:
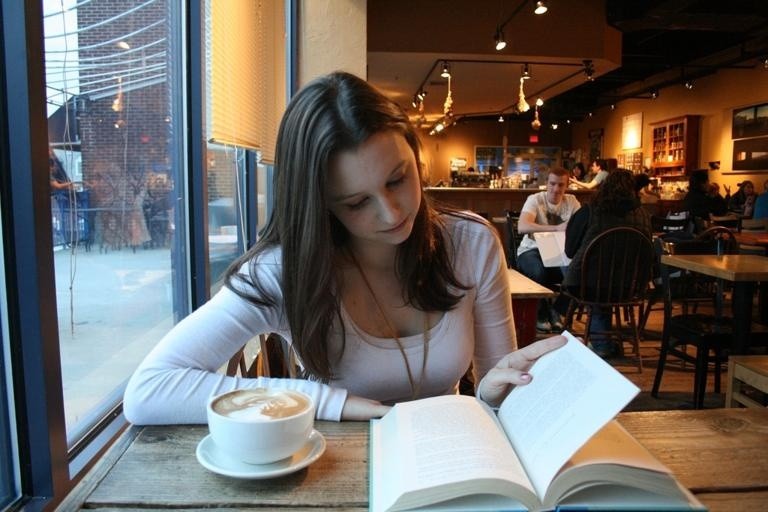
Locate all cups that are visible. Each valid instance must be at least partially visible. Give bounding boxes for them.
[207,387,313,464]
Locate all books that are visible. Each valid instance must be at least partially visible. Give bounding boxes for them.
[533,232,571,267]
[368,328,708,512]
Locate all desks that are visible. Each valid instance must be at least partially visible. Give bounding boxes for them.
[502,267,557,348]
[722,351,767,405]
[82,402,768,510]
[664,249,767,379]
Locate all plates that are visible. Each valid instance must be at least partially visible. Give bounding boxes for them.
[195,429,327,480]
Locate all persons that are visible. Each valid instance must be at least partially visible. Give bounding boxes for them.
[122,71,567,427]
[564,168,653,359]
[49,160,71,195]
[515,165,582,333]
[567,158,767,229]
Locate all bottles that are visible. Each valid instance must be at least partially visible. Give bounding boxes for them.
[725,190,731,210]
[658,152,662,163]
[716,237,724,256]
[669,124,683,161]
[489,173,500,189]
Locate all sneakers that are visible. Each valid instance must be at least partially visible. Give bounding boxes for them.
[550,321,564,329]
[536,318,552,331]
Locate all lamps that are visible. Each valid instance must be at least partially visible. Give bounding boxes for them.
[414,0,599,135]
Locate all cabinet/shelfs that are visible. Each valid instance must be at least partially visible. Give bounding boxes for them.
[647,114,699,180]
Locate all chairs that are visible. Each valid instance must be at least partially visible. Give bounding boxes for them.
[651,239,736,404]
[504,210,527,271]
[563,228,654,377]
